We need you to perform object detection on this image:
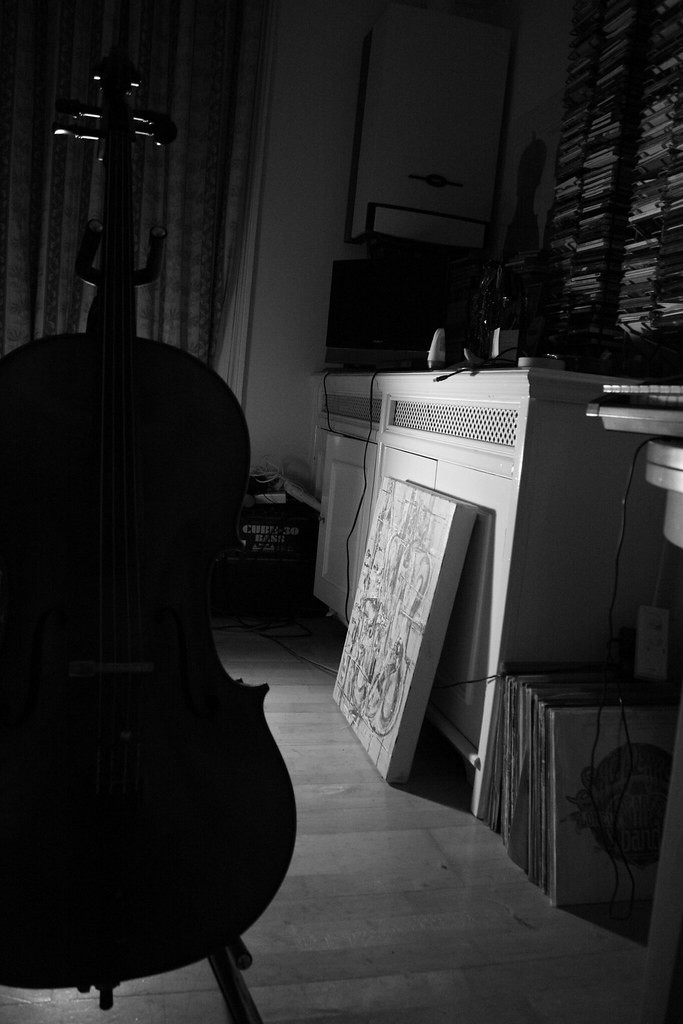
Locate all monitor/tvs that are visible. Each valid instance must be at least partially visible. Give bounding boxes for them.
[325,257,450,370]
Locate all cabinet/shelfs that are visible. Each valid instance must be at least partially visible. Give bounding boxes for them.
[536,0,683,363]
[315,372,682,818]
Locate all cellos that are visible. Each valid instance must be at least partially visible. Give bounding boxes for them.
[0,53,297,1012]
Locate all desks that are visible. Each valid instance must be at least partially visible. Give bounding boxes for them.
[635,435,682,1024]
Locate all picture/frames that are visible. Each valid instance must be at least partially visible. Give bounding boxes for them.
[343,0,516,250]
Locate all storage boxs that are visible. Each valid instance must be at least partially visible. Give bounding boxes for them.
[226,505,317,564]
